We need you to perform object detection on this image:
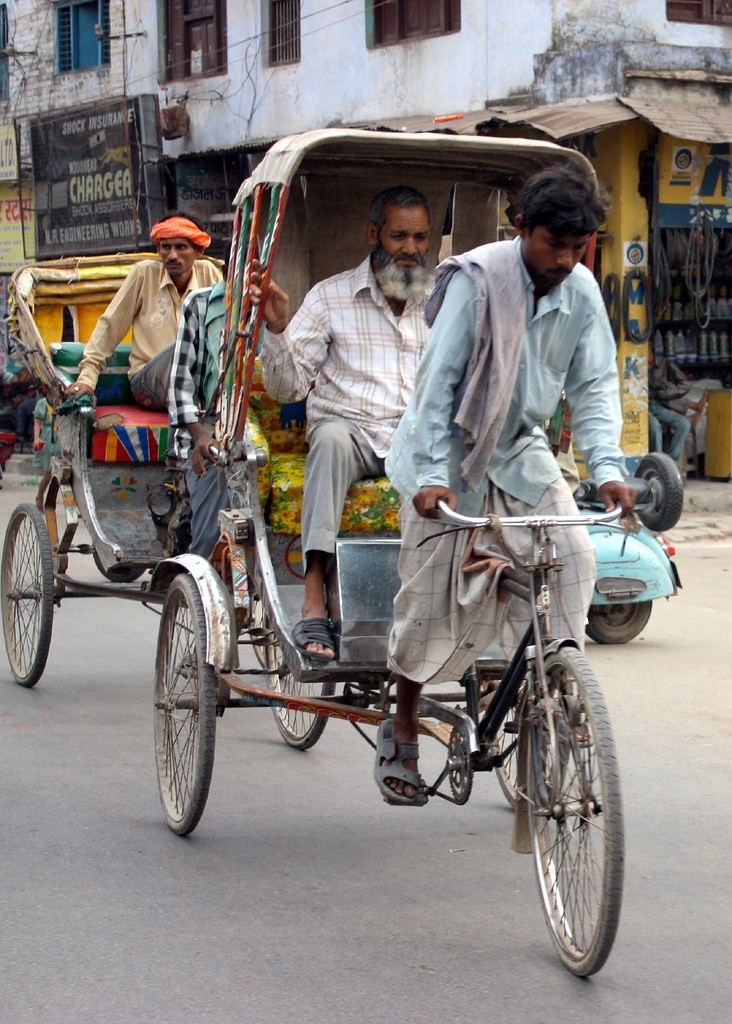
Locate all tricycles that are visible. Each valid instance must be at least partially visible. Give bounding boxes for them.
[0,252,336,752]
[152,122,644,979]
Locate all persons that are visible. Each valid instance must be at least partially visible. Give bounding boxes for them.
[249,184,439,662]
[61,211,228,563]
[0,388,55,513]
[648,359,696,472]
[374,166,639,808]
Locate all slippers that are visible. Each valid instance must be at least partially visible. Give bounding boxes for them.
[374,719,421,803]
[292,617,335,660]
[540,707,593,748]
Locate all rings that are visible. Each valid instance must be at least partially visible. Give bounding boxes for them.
[75,387,78,391]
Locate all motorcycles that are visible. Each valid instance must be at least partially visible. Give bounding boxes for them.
[586,517,683,645]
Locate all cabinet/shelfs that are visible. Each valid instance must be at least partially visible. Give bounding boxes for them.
[704,389,732,481]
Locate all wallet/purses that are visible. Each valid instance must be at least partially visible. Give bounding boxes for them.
[33,441,45,451]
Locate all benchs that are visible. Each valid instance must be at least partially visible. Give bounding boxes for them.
[235,376,400,540]
[51,340,170,463]
